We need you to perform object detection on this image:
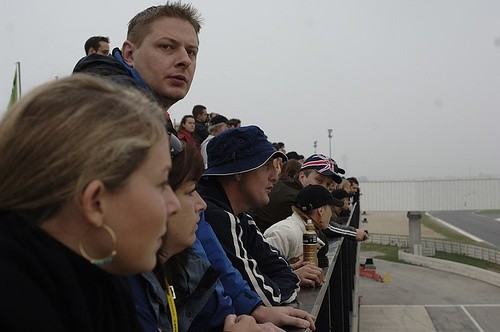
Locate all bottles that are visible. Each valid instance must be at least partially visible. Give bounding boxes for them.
[303,224,318,267]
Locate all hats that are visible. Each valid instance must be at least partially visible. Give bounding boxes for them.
[208,114,229,126]
[299,154,342,184]
[202,124,288,175]
[295,184,344,211]
[328,157,345,175]
[285,151,304,161]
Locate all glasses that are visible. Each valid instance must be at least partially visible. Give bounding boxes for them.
[168,131,184,158]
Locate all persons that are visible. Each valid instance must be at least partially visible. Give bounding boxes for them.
[262,185,350,268]
[68,1,316,332]
[198,125,301,308]
[141,134,283,332]
[179,105,371,243]
[84,36,110,57]
[1,72,183,332]
[246,153,335,234]
